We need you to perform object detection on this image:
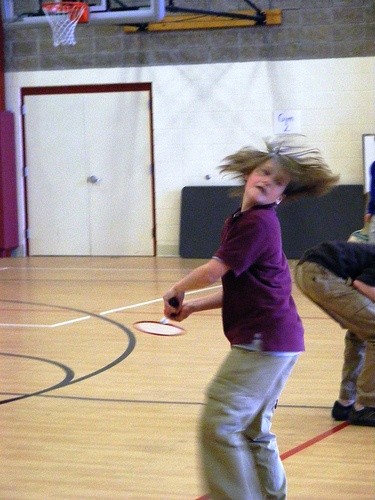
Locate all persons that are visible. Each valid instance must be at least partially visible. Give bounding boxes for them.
[364,135,375,244]
[293,240,375,428]
[161,131,341,500]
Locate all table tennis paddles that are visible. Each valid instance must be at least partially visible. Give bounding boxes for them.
[131,296,187,338]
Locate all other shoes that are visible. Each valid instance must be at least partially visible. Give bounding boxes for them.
[347,406,375,428]
[331,401,357,420]
